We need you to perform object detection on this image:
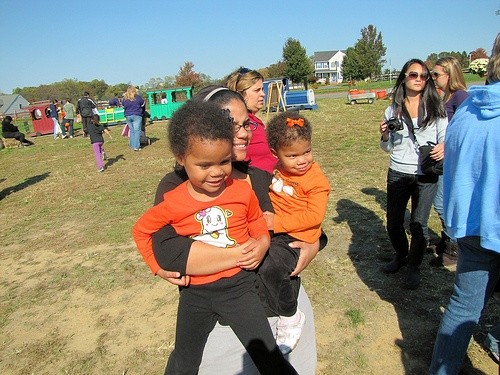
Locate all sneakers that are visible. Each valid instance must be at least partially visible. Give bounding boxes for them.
[264,309,306,355]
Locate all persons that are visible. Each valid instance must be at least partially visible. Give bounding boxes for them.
[122,86,151,150]
[45,99,62,140]
[132,84,331,375]
[379,59,448,291]
[432,31,500,375]
[60,99,76,139]
[82,114,112,173]
[75,91,97,137]
[222,67,279,175]
[1,116,34,146]
[422,56,468,268]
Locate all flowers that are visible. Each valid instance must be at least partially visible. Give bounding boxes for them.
[468,57,489,78]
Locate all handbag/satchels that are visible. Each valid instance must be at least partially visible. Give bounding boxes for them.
[419,141,443,176]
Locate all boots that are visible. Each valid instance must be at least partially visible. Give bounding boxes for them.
[432,239,458,266]
[426,231,449,253]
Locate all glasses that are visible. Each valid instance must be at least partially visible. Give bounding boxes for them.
[405,72,430,81]
[236,68,253,86]
[232,120,258,133]
[431,72,450,77]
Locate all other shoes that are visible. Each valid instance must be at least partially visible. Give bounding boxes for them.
[55,137,59,140]
[384,257,407,273]
[102,152,106,161]
[134,148,141,151]
[62,135,66,139]
[405,269,420,289]
[98,167,104,173]
[482,339,500,361]
[70,135,73,138]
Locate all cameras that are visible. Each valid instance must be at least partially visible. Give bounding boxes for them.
[383,118,401,132]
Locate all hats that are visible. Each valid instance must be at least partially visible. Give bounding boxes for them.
[5,116,13,121]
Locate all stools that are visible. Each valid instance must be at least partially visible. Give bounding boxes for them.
[2,138,19,149]
